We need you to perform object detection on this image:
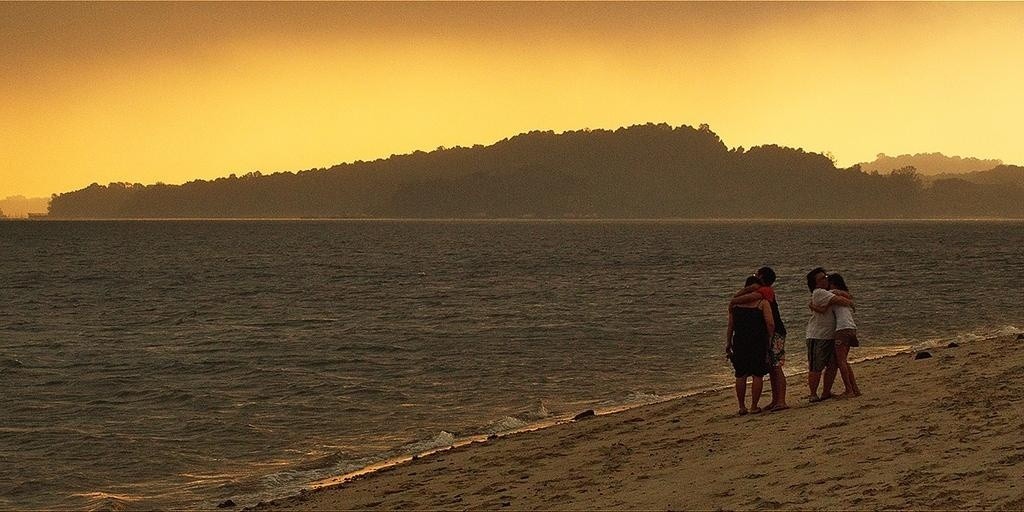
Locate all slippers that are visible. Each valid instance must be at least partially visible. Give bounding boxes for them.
[739,400,789,415]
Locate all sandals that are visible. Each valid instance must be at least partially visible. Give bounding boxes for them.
[809,389,861,402]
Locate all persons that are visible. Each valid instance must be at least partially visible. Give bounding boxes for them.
[726,276,776,415]
[806,268,856,402]
[808,273,861,398]
[731,266,791,412]
